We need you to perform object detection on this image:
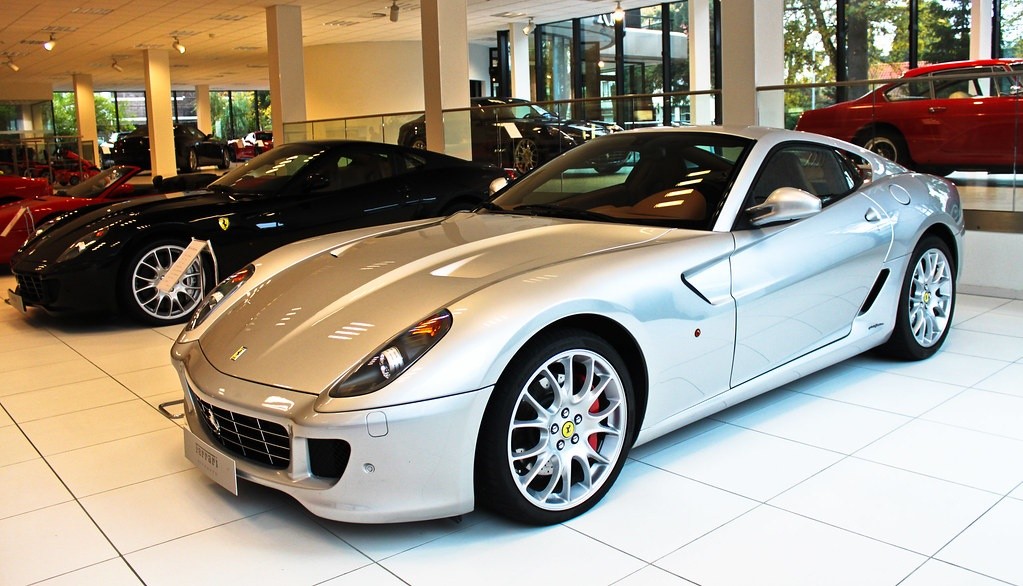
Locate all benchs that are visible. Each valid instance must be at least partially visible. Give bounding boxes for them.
[163,174,217,192]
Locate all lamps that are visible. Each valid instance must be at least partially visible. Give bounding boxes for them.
[171,36,185,54]
[390,0,399,21]
[6,56,19,72]
[523,17,536,35]
[111,58,123,73]
[43,32,56,51]
[614,0,625,21]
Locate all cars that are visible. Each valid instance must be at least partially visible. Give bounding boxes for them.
[793,58,1023,176]
[98,132,122,163]
[0,172,53,206]
[119,123,233,173]
[397,96,632,177]
[53,151,110,188]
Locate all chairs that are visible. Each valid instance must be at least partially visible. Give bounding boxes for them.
[751,150,818,203]
[314,166,378,192]
[949,91,971,98]
[615,152,706,221]
[152,175,163,195]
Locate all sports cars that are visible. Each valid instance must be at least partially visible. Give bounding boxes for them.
[227,131,273,162]
[169,125,969,529]
[7,140,510,327]
[0,165,152,274]
